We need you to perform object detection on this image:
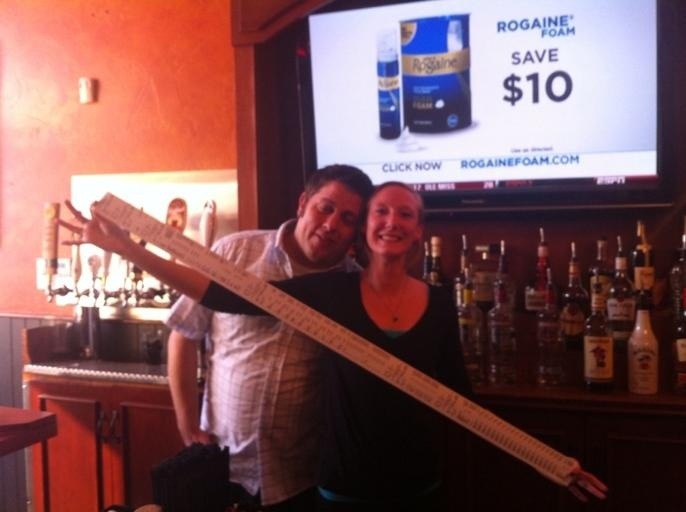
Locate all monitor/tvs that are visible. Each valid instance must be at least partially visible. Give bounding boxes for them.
[293,0,685,215]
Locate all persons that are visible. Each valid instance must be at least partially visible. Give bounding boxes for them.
[159,163,372,512]
[55,180,469,511]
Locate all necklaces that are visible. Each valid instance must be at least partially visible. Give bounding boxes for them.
[366,277,410,323]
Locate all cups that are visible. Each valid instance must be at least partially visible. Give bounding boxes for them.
[446,20,462,54]
[72,307,103,363]
[141,328,163,363]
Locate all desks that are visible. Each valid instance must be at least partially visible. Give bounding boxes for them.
[0,404,60,458]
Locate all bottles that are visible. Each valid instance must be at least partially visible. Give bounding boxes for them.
[421,220,685,393]
[375,21,400,139]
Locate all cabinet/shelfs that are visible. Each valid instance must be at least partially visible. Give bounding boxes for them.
[22,357,686,512]
